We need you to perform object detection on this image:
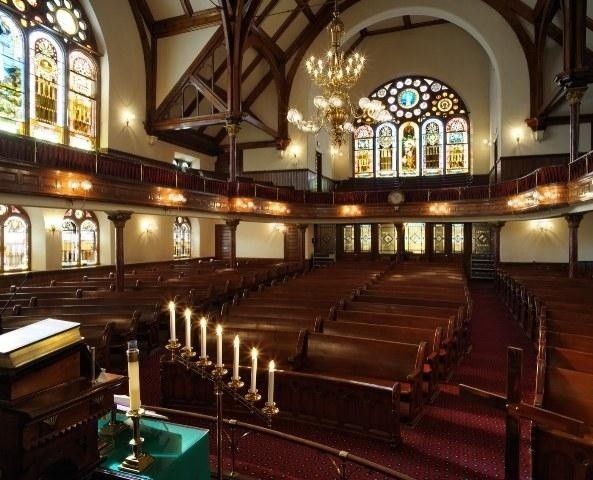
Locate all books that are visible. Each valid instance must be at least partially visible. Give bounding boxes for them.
[0,317,83,369]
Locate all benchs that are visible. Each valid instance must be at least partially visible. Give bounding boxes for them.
[1,255,471,449]
[493,261,591,450]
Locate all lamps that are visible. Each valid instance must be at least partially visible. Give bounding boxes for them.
[284,0,394,159]
